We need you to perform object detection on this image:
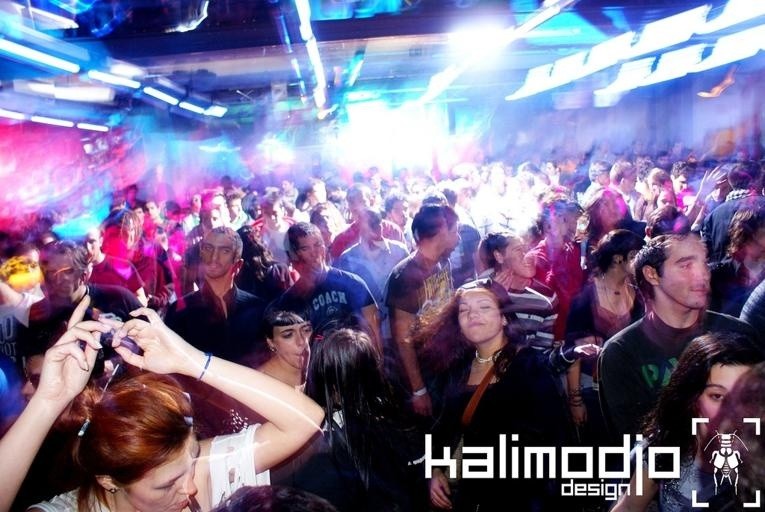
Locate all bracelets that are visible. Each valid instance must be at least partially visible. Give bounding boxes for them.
[412,385,428,398]
[193,351,214,382]
[565,385,586,410]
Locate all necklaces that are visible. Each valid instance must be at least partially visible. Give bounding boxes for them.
[473,348,504,367]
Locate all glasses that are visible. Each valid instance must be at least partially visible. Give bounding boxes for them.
[20,369,41,390]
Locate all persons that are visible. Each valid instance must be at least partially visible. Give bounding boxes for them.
[416,278,581,511]
[705,203,765,318]
[607,331,763,511]
[519,197,594,341]
[254,301,314,393]
[20,335,61,405]
[567,227,646,348]
[12,239,144,342]
[163,226,274,366]
[669,160,698,209]
[268,223,385,374]
[88,209,160,319]
[577,162,726,271]
[155,156,579,227]
[232,227,293,301]
[693,159,765,264]
[330,208,409,309]
[606,159,645,222]
[0,294,321,510]
[580,187,647,274]
[594,231,740,440]
[687,162,734,232]
[296,326,426,512]
[477,228,603,374]
[381,204,462,415]
[583,158,613,209]
[634,168,677,219]
[641,205,694,245]
[644,188,690,235]
